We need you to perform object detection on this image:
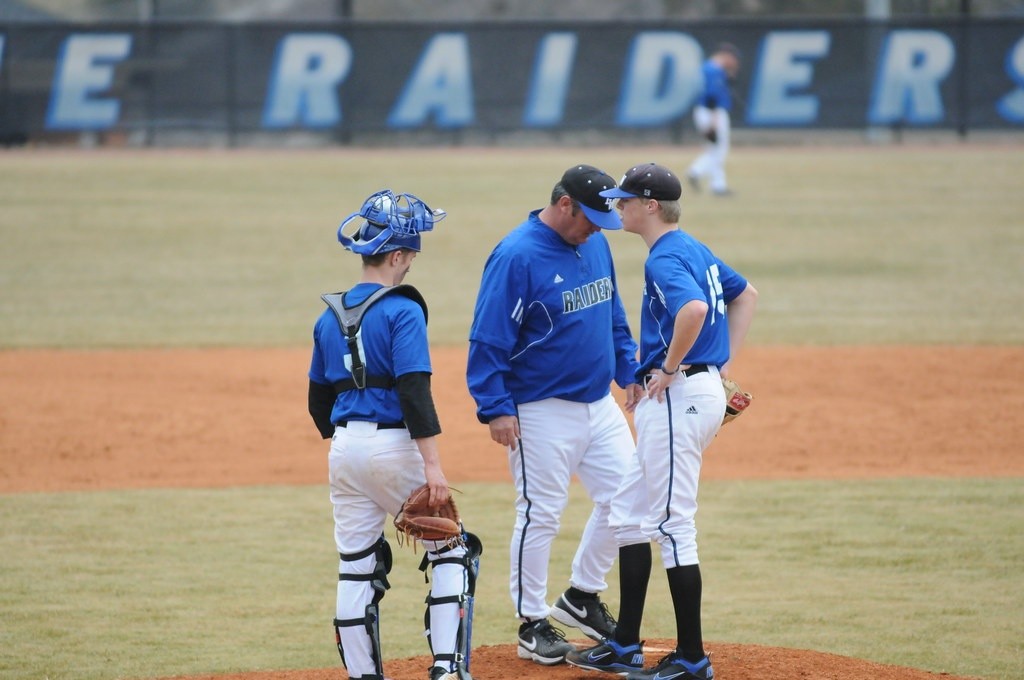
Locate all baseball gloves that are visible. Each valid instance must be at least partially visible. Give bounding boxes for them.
[391,482,470,554]
[722,379,753,426]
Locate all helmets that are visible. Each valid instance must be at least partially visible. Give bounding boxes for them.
[359,220,421,254]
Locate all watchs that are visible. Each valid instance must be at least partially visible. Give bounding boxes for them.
[660,359,680,375]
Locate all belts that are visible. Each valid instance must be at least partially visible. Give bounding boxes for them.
[639,364,720,392]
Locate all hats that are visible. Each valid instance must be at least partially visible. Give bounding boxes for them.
[560,164,623,230]
[599,162,682,201]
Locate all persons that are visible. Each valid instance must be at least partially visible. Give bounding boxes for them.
[467,165,645,665]
[566,162,758,680]
[685,42,738,196]
[307,191,483,680]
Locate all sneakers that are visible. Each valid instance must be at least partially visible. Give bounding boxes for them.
[428,666,460,680]
[517,619,574,666]
[550,590,618,643]
[626,649,714,680]
[565,638,646,677]
